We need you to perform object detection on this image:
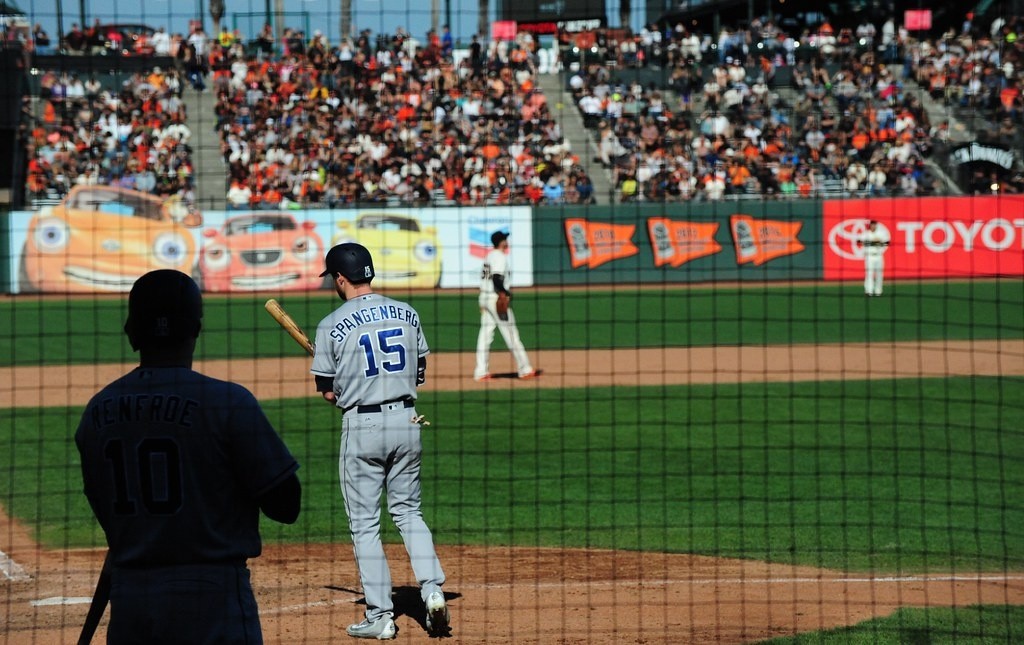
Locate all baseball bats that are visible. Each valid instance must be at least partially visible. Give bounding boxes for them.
[264,299,315,358]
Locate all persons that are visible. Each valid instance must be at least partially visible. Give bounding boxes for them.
[312,243,451,639]
[1,1,1023,206]
[74,269,301,645]
[473,232,544,381]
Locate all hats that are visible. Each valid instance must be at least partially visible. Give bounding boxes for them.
[491,231,510,245]
[123,269,204,321]
[319,242,375,283]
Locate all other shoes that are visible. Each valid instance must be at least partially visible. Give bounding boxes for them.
[518,368,545,380]
[474,373,499,382]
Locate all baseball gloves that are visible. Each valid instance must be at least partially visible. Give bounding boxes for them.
[497,294,510,321]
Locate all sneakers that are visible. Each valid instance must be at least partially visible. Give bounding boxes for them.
[425,591,451,635]
[345,617,395,641]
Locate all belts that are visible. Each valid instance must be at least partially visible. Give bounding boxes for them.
[357,400,414,414]
